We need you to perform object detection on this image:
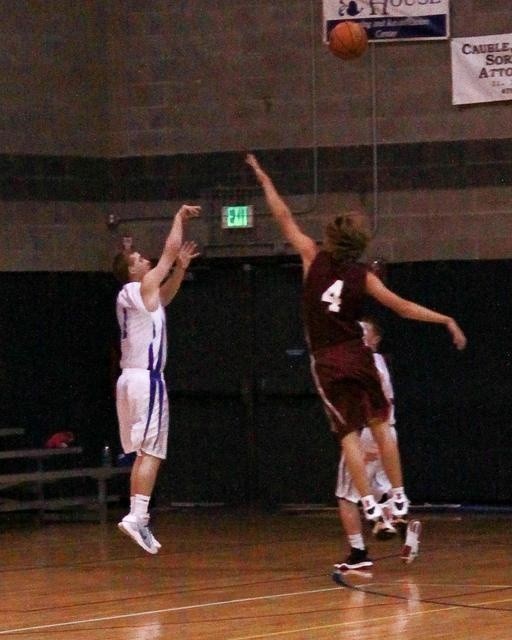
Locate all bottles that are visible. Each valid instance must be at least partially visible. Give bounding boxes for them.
[102,446,112,468]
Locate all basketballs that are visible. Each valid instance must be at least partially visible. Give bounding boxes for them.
[329,21,367,60]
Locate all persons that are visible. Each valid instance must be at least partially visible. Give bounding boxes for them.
[111,204,203,555]
[243,151,470,545]
[331,314,424,570]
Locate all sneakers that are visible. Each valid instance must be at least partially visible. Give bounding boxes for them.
[334,501,422,569]
[118,514,162,555]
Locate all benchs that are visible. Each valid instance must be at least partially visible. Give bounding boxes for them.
[1,426,140,523]
